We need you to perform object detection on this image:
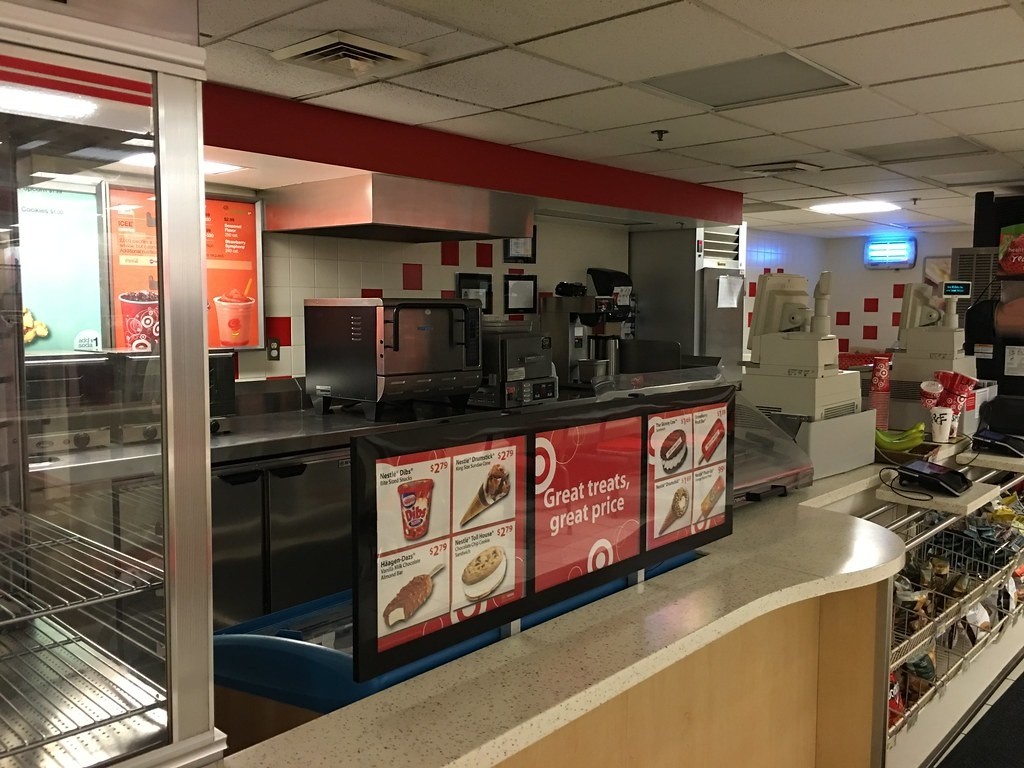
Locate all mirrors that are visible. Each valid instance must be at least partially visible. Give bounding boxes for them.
[922,256,952,295]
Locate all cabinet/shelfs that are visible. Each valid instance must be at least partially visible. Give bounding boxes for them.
[27,447,351,664]
[1,0,230,768]
[860,465,1024,739]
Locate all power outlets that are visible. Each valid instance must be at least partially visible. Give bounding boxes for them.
[267,337,279,360]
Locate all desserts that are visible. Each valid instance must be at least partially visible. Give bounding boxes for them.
[660,429,687,473]
[462,547,506,601]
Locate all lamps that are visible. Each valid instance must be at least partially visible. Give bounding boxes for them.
[862,236,918,270]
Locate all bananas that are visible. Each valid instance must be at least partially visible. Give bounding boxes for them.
[875,419,926,453]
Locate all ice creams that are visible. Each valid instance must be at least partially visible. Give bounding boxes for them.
[658,487,689,535]
[460,464,510,526]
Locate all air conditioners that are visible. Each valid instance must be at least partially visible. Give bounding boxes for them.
[631,221,747,387]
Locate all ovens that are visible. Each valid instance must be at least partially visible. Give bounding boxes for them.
[302,297,484,421]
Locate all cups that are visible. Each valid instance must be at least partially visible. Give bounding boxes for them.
[933,370,979,438]
[118,292,160,351]
[919,381,944,409]
[930,407,952,442]
[868,356,889,431]
[398,478,435,541]
[213,296,255,348]
[578,359,607,383]
